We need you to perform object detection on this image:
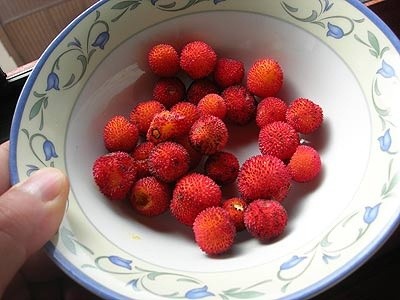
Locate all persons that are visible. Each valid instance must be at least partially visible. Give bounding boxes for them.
[0,140,103,300]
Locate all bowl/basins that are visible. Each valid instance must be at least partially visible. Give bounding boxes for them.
[9,1,399,300]
